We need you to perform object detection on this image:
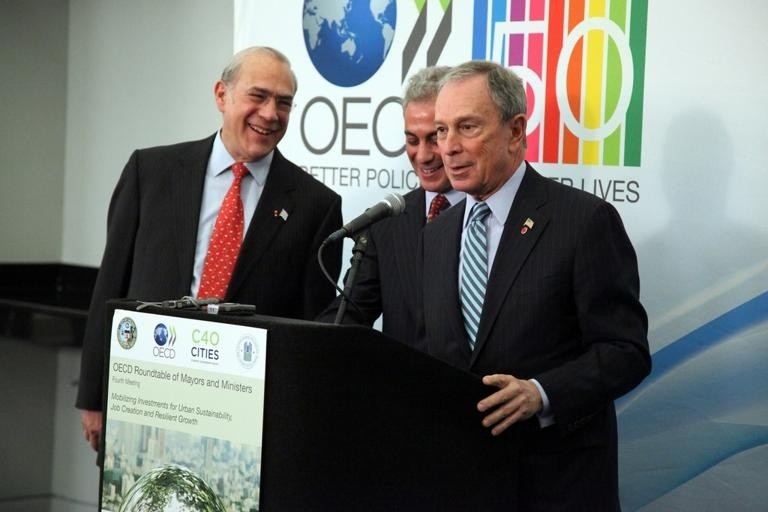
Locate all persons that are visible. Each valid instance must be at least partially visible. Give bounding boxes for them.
[313,66,467,353]
[414,61,651,511]
[75,48,343,512]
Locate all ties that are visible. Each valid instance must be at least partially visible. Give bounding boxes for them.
[197,162,250,310]
[459,202,492,351]
[427,193,451,222]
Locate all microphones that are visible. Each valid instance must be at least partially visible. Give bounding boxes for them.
[327,192,406,241]
